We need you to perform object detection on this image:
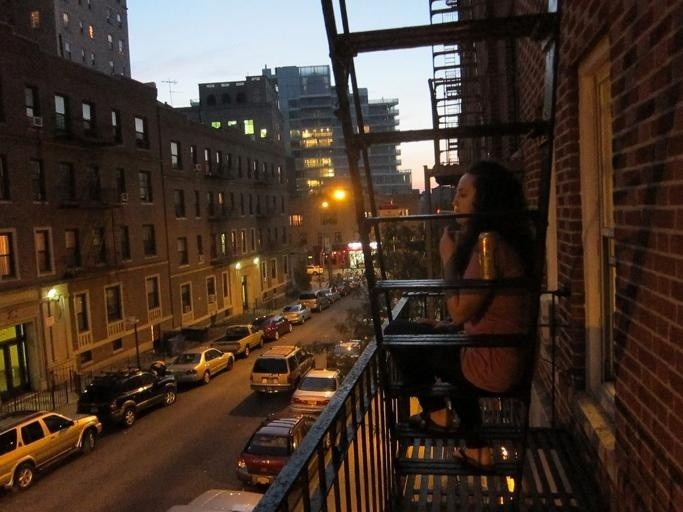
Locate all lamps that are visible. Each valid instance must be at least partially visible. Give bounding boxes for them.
[43,289,65,319]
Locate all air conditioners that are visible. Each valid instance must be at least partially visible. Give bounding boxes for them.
[194,163,203,174]
[121,192,128,202]
[29,116,43,129]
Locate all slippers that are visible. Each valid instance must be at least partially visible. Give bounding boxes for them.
[448,446,494,471]
[407,410,450,433]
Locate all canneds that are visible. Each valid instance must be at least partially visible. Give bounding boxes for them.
[478,231,496,281]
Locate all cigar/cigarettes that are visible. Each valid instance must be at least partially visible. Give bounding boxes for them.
[435,208,458,220]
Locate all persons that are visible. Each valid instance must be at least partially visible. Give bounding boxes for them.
[380,157,542,477]
